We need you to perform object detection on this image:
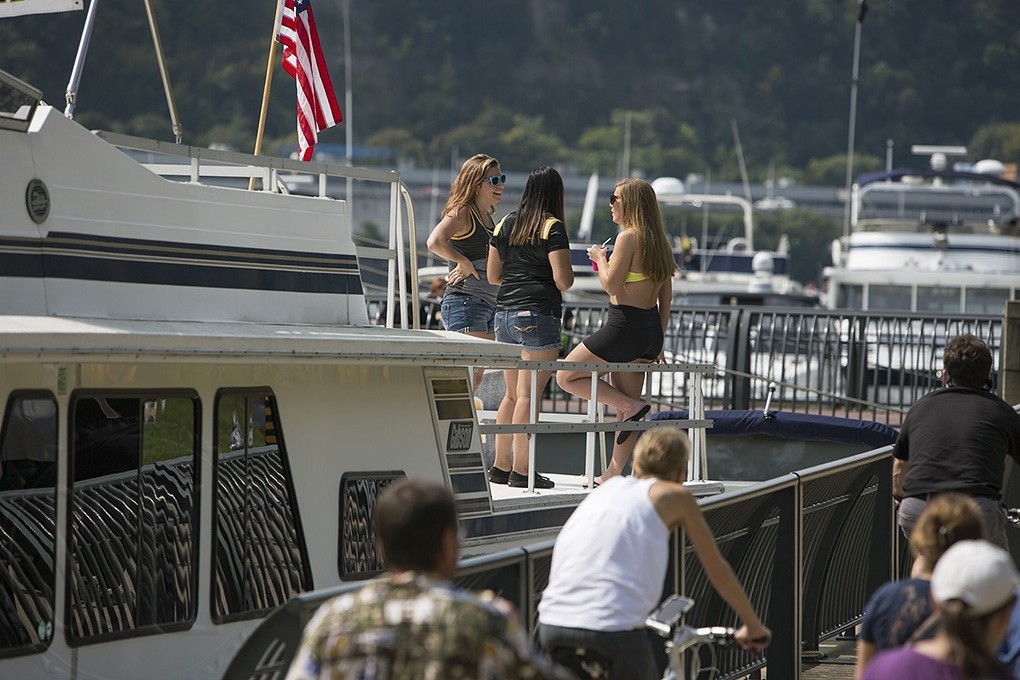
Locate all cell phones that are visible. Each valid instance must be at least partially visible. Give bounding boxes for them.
[649,595,694,627]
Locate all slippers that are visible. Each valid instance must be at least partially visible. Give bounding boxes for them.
[616,405,652,445]
[583,480,600,488]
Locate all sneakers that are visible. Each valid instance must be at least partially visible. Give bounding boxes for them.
[508,470,555,489]
[488,465,513,484]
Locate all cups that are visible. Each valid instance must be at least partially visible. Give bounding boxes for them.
[592,248,605,271]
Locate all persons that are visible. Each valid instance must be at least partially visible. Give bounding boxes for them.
[418,277,449,331]
[286,479,583,680]
[427,154,506,395]
[855,493,1020,680]
[377,265,428,325]
[485,167,575,491]
[556,176,673,492]
[890,332,1020,560]
[533,425,774,680]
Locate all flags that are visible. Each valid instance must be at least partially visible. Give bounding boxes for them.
[277,1,342,161]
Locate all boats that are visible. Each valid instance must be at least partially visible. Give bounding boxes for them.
[1,2,1020,680]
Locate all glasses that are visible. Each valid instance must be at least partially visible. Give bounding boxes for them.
[483,174,506,186]
[610,194,622,205]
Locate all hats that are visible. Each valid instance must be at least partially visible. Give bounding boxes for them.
[929,538,1020,616]
